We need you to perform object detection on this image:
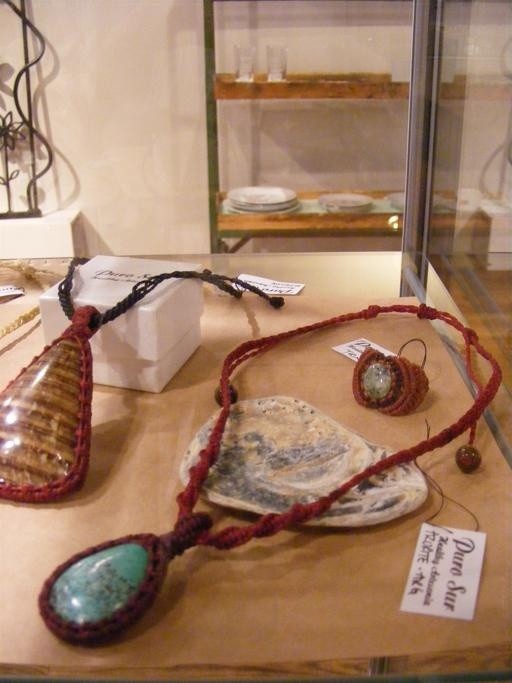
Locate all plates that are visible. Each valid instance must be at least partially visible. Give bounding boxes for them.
[442,198,497,211]
[380,191,438,212]
[220,185,304,218]
[316,193,371,215]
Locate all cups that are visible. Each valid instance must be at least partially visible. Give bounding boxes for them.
[264,41,287,83]
[454,186,483,209]
[232,43,258,84]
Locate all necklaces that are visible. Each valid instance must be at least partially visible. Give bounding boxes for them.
[38,302,503,644]
[1,253,284,502]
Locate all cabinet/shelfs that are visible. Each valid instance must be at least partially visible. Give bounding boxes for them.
[202,0,512,258]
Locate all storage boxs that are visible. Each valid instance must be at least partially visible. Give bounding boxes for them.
[36,254,211,396]
